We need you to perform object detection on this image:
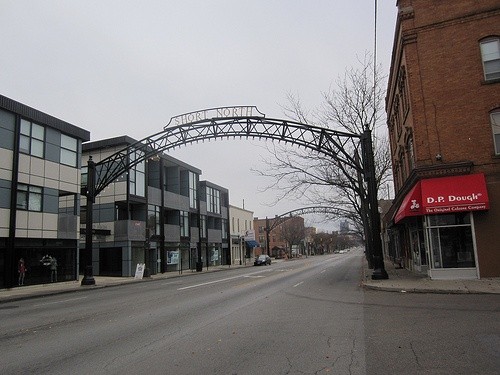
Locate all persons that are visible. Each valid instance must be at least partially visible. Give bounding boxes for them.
[18,258,27,286]
[51,257,58,282]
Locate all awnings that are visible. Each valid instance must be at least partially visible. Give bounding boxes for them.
[246,240,261,248]
[394,174,490,223]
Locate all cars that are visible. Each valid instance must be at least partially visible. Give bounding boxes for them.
[334,248,351,254]
[254,254,272,266]
